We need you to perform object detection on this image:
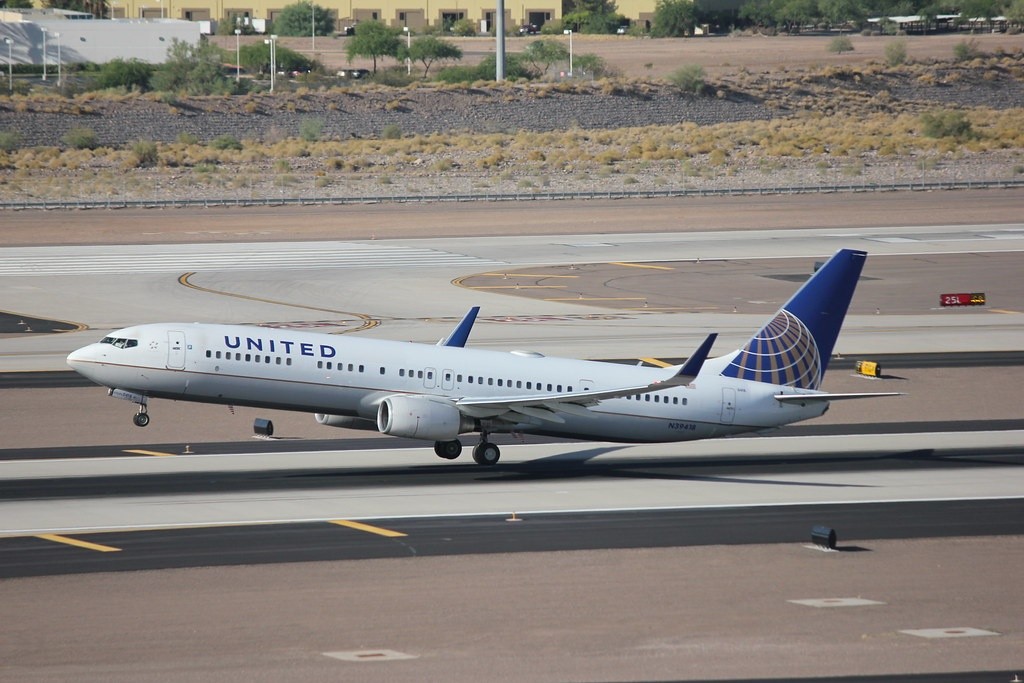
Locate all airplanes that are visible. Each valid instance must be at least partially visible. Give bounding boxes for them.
[64,247,909,472]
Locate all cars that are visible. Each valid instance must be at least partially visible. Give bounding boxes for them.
[519,24,536,34]
[616,26,629,34]
[275,69,371,79]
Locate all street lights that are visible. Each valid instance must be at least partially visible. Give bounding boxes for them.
[271,35,277,84]
[564,29,572,75]
[265,39,273,92]
[403,27,411,74]
[5,40,13,92]
[309,2,315,51]
[53,31,61,84]
[234,30,241,82]
[40,27,48,80]
